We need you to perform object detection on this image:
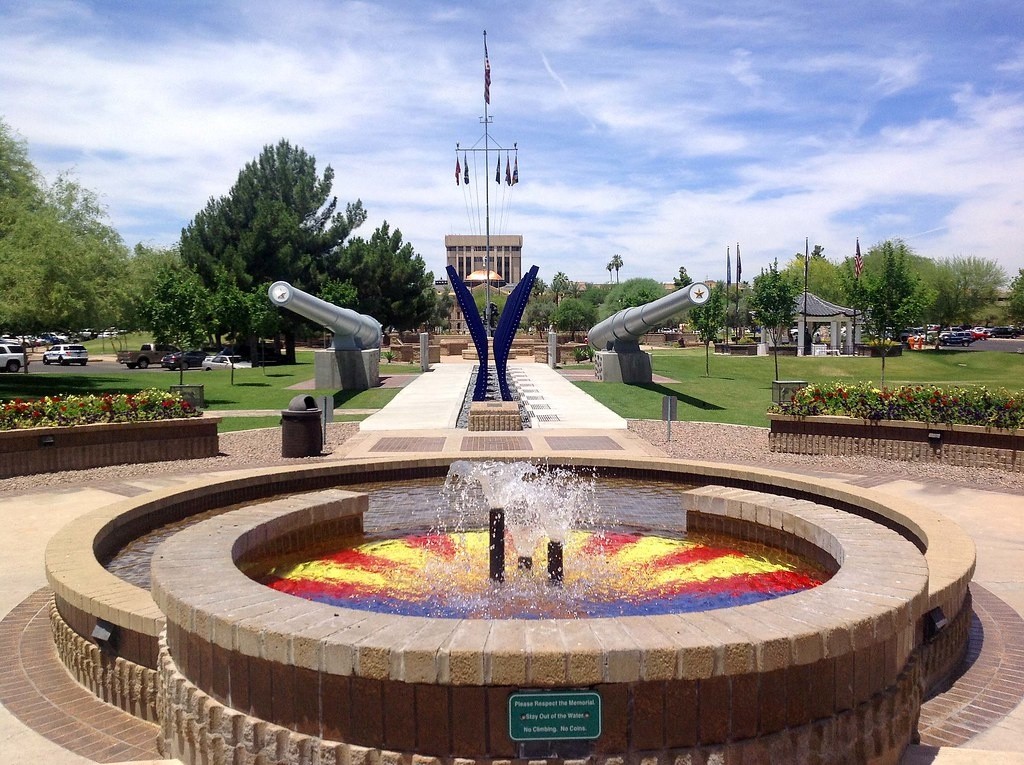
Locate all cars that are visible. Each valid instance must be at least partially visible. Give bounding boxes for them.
[1,328,128,347]
[788,323,1022,349]
[160,350,210,370]
[200,356,261,371]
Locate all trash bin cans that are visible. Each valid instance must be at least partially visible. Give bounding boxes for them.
[280,393,322,458]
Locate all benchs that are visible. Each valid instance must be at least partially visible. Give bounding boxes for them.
[817,349,841,357]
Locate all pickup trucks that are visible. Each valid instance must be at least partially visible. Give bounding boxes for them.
[117,343,182,369]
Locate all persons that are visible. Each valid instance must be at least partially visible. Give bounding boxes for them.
[908,334,915,350]
[788,330,798,343]
[815,333,821,344]
[916,333,924,350]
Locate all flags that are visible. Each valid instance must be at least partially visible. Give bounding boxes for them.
[505,151,511,185]
[737,246,742,282]
[727,248,732,285]
[464,154,471,185]
[455,154,462,186]
[855,239,864,279]
[496,150,501,184]
[511,149,519,186]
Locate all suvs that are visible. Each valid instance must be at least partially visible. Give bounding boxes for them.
[42,344,89,365]
[0,342,30,373]
